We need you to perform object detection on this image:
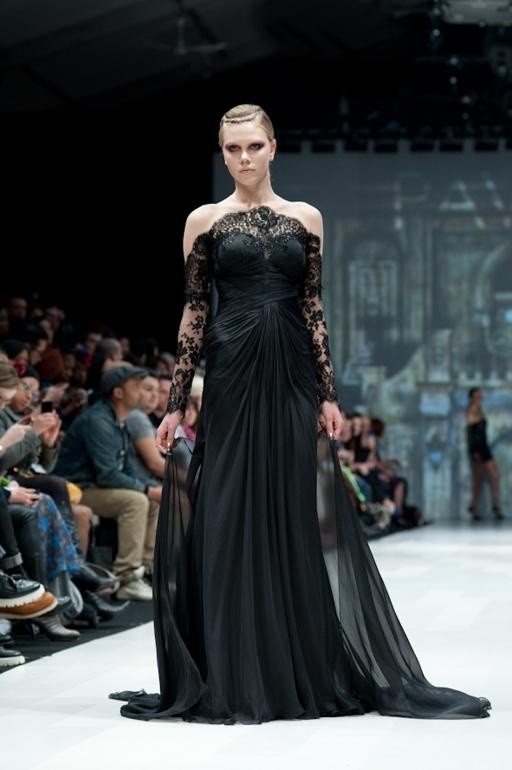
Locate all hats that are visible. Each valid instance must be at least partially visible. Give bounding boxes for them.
[104,367,146,392]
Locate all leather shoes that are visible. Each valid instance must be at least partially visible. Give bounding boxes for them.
[0,634,26,666]
[87,590,128,622]
[75,566,117,590]
[1,571,44,609]
[1,593,57,619]
[467,506,503,521]
[35,614,79,641]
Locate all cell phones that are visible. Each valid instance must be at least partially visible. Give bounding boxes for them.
[41,401,53,413]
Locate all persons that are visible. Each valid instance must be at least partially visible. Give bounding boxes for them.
[0,287,205,668]
[319,409,412,537]
[107,101,491,727]
[466,387,505,522]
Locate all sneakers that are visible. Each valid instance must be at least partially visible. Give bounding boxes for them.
[119,579,152,600]
